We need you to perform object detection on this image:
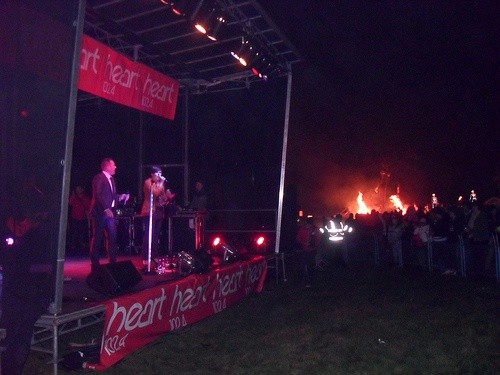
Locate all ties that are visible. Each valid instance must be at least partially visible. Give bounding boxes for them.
[110,178,114,195]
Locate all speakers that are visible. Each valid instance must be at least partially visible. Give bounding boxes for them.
[87,260,142,298]
[177,247,214,275]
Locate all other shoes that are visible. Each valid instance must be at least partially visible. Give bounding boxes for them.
[305,279,311,287]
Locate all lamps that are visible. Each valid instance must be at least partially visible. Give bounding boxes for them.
[231,38,274,80]
[213,236,246,264]
[160,0,229,41]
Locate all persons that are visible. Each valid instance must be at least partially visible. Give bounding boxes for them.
[88,158,130,266]
[190,180,211,209]
[139,166,176,257]
[69,184,90,253]
[295,201,500,288]
